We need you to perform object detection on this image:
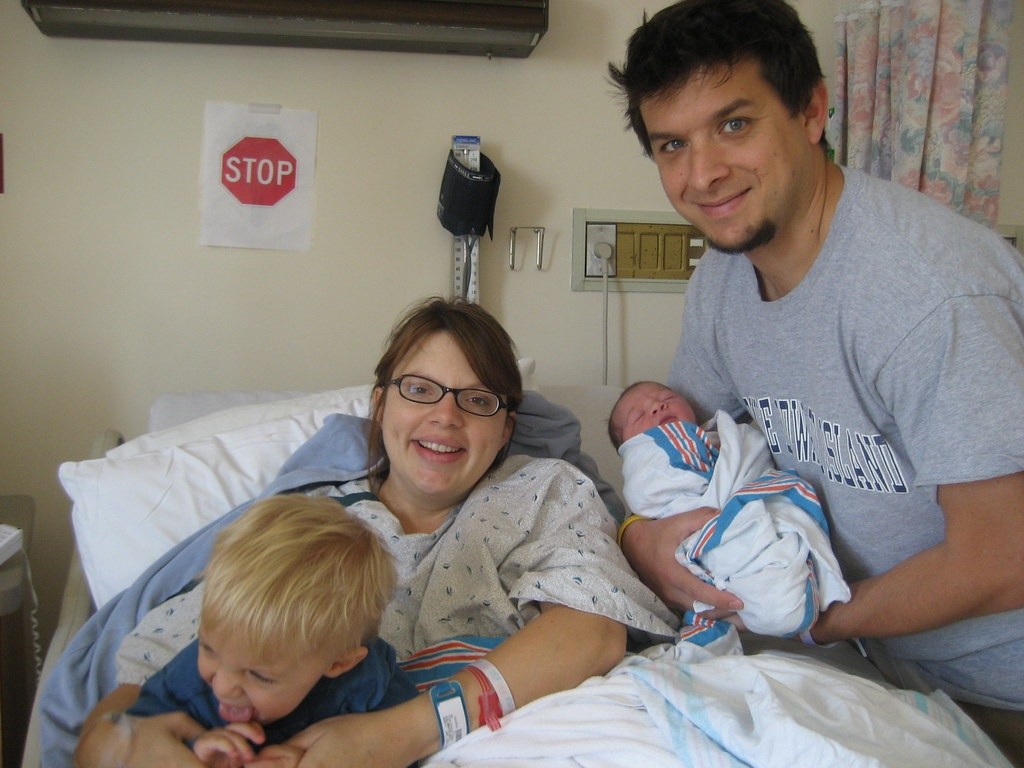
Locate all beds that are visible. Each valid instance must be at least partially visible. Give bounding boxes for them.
[21,392,1017,768]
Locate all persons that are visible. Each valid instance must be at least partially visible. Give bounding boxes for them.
[609,381,852,639]
[608,0,1024,768]
[73,296,678,768]
[127,494,421,768]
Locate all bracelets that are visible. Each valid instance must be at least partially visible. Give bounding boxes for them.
[616,514,653,549]
[426,659,516,751]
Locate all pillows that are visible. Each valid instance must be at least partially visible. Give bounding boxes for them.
[59,359,538,611]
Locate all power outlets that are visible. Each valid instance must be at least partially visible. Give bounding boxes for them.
[586,223,618,278]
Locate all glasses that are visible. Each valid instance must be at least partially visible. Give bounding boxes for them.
[381,374,513,417]
